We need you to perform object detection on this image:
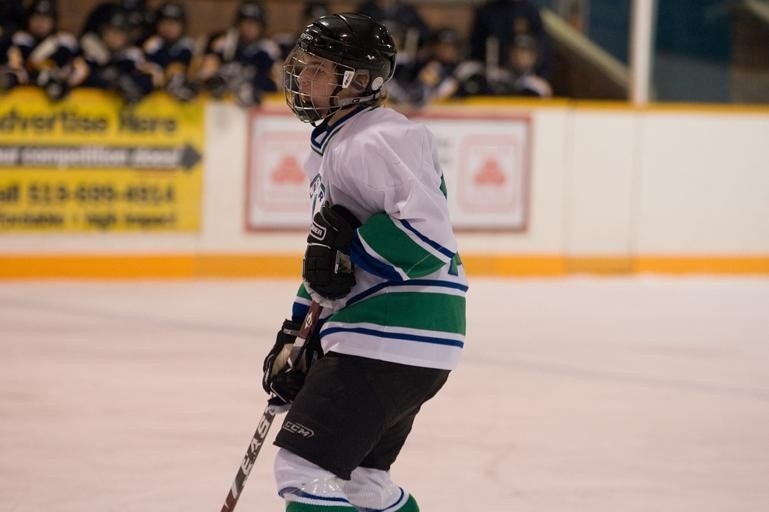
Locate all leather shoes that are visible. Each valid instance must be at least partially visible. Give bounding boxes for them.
[261,317,308,408]
[301,199,358,302]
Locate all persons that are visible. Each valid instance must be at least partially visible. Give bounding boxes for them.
[376,1,555,102]
[0,1,333,112]
[263,8,468,511]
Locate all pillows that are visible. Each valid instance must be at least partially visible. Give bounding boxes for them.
[298,11,398,99]
